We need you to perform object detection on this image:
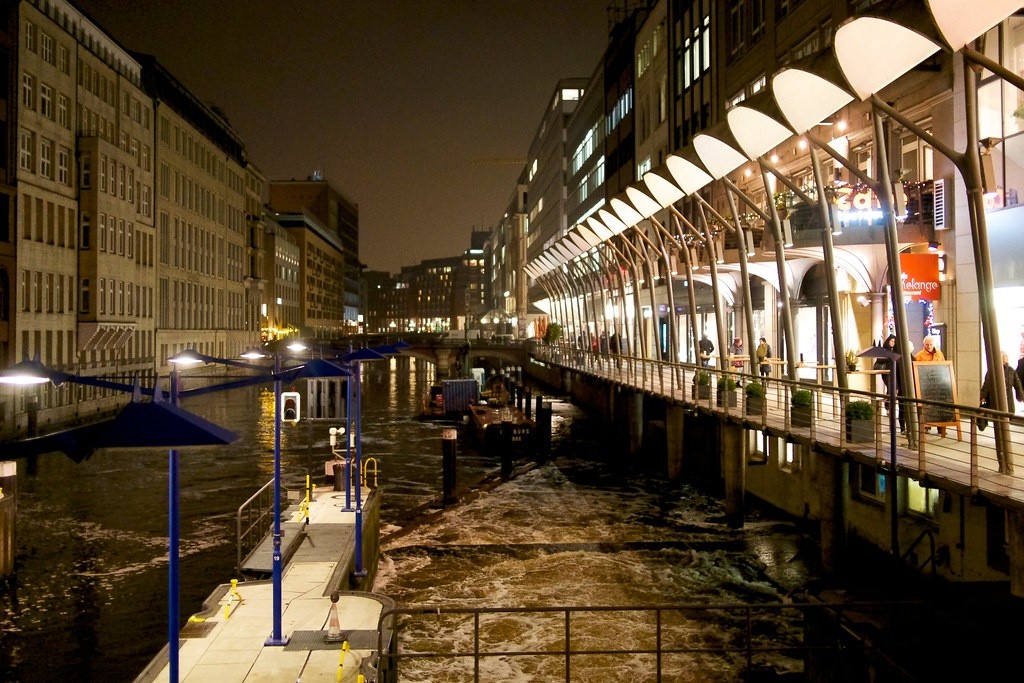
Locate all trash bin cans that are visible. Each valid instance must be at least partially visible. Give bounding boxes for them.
[333,464,346,491]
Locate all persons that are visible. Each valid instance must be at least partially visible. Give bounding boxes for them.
[757,338,772,387]
[730,338,744,387]
[916,336,948,435]
[898,341,917,435]
[877,334,899,414]
[570,331,623,368]
[979,350,1024,422]
[699,334,714,367]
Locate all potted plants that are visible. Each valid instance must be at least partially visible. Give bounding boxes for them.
[746,382,767,416]
[574,349,586,365]
[790,390,812,427]
[845,349,859,371]
[691,371,709,399]
[717,377,737,407]
[845,399,874,444]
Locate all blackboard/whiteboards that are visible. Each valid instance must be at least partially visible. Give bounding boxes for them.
[912,361,961,426]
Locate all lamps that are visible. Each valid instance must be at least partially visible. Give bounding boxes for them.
[857,296,872,307]
[715,241,724,264]
[978,131,1024,156]
[746,231,755,257]
[653,261,660,280]
[928,241,942,252]
[624,270,631,287]
[783,219,793,248]
[638,266,645,284]
[671,256,677,276]
[691,248,699,271]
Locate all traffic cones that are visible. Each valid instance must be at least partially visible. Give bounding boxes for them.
[324,602,348,642]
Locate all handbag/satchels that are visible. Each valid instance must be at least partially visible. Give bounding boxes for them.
[976,400,988,432]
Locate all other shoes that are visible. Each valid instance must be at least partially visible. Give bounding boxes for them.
[736,383,743,388]
[901,429,908,434]
[939,430,947,434]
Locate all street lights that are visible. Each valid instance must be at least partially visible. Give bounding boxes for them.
[0,359,354,683]
[165,338,412,647]
